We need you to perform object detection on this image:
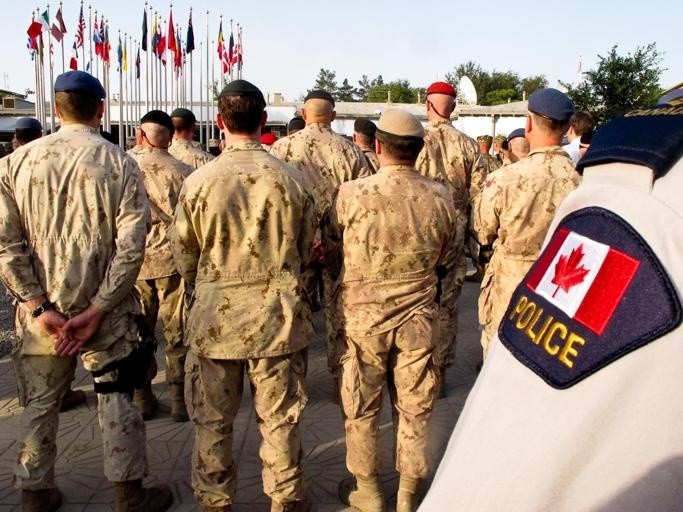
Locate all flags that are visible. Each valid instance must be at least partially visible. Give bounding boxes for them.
[27,4,243,78]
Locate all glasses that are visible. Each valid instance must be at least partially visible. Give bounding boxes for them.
[579,145,586,149]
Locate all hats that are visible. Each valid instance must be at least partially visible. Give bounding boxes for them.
[288,118,305,131]
[260,132,276,144]
[170,108,196,122]
[54,71,106,100]
[493,135,507,143]
[427,82,458,100]
[16,118,42,130]
[208,139,219,147]
[528,88,574,121]
[304,90,336,108]
[377,107,426,136]
[508,128,525,141]
[219,80,266,109]
[477,135,493,144]
[354,118,377,138]
[140,110,175,133]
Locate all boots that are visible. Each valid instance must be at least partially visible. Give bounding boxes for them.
[21,488,63,512]
[394,474,421,512]
[130,379,158,421]
[114,478,174,512]
[59,389,86,413]
[338,469,385,512]
[464,267,483,284]
[270,490,312,512]
[168,382,190,422]
